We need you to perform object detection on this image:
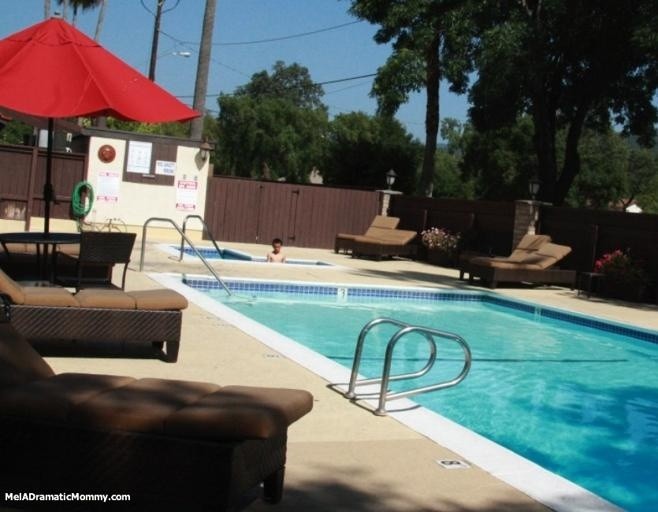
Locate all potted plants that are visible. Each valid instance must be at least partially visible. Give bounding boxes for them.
[459,230,485,268]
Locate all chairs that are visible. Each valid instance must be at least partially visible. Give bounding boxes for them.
[459,233,572,289]
[334,215,417,261]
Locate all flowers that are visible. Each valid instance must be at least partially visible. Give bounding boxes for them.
[420,227,461,258]
[592,250,643,287]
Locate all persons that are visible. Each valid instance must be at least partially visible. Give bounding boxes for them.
[265,238,288,263]
[625,199,643,213]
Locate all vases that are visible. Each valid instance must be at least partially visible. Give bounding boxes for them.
[615,281,632,297]
[434,251,457,267]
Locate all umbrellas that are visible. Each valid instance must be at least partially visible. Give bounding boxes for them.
[2,11,203,233]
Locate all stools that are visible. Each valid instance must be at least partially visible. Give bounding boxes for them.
[578,272,608,299]
